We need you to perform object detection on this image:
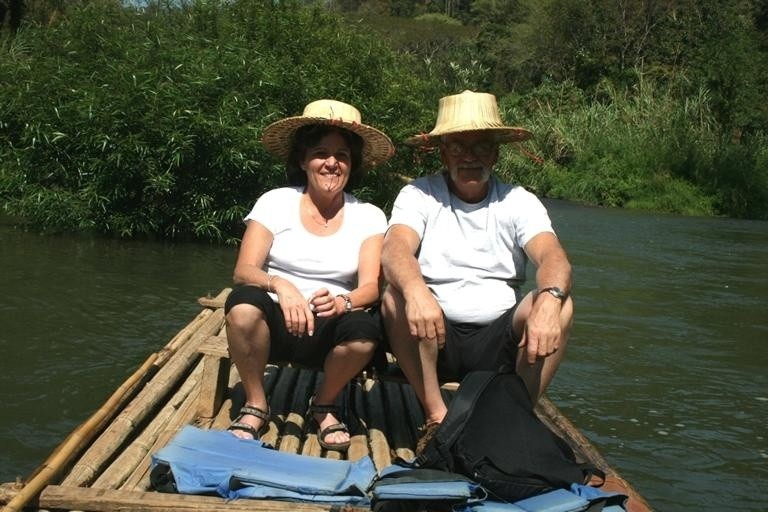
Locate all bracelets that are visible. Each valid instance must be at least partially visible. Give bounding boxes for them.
[268,274,277,293]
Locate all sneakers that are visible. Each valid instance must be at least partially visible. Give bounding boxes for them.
[416,420,440,456]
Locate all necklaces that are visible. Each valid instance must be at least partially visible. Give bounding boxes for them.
[310,211,330,229]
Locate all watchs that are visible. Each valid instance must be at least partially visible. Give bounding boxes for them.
[538,287,564,298]
[336,295,352,313]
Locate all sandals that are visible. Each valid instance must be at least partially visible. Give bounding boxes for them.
[228,404,271,440]
[306,397,350,451]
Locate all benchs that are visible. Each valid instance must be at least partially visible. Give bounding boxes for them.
[197,335,460,418]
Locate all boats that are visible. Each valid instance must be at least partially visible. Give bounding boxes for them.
[0,289,655,511]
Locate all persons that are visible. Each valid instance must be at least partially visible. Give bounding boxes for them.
[380,90,574,456]
[224,99,396,451]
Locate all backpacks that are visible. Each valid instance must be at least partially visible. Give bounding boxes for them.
[412,364,604,504]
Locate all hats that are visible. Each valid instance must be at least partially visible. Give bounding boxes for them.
[404,88,534,147]
[262,100,396,165]
